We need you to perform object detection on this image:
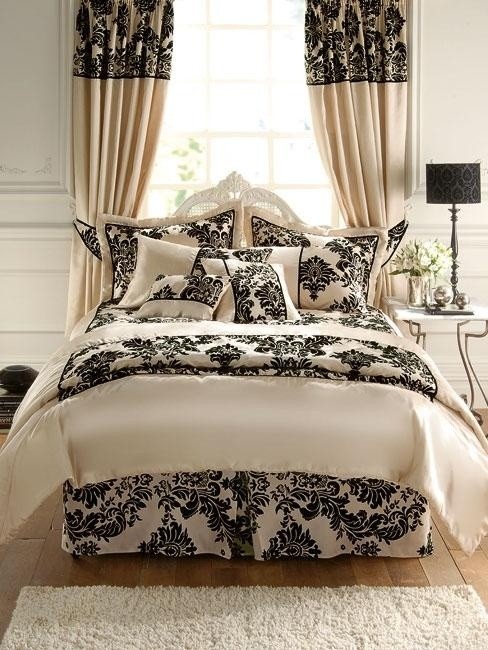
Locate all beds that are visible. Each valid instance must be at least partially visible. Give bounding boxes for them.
[0,168,488,563]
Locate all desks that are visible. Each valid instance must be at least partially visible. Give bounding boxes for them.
[381,296,488,439]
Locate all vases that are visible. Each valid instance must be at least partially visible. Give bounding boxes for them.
[404,274,438,309]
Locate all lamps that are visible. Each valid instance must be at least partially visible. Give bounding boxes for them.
[425,162,482,316]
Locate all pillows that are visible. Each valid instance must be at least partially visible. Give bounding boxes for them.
[247,245,370,316]
[245,205,390,307]
[197,257,304,323]
[95,197,245,305]
[133,273,235,320]
[117,233,275,308]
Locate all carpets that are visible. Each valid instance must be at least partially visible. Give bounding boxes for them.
[0,582,487,650]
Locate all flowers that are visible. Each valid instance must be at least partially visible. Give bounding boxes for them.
[386,238,453,278]
